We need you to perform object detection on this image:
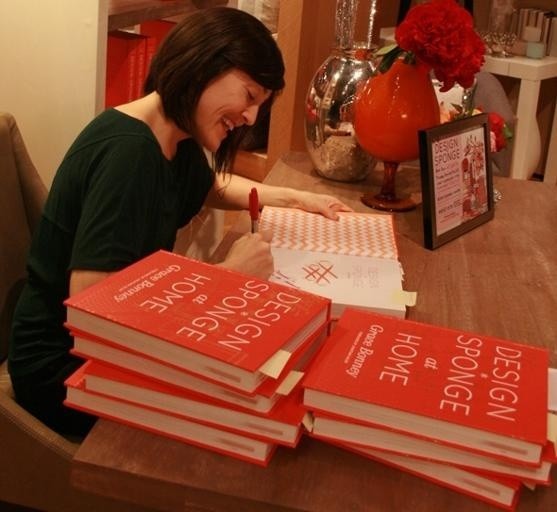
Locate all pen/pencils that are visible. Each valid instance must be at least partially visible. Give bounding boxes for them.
[248,188,260,233]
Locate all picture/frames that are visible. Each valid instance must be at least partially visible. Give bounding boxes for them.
[417,112,494,250]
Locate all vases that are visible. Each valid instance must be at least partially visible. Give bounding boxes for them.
[352,60,439,213]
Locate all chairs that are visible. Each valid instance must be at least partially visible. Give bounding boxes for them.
[0,110,154,511]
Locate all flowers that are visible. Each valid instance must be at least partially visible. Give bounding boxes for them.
[365,1,488,94]
[440,100,513,154]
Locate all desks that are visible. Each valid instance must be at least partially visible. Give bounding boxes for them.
[71,151,557,511]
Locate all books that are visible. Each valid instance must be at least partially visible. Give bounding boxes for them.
[254,201,419,323]
[304,306,557,512]
[63,252,332,468]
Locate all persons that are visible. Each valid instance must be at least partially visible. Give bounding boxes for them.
[7,6,358,445]
